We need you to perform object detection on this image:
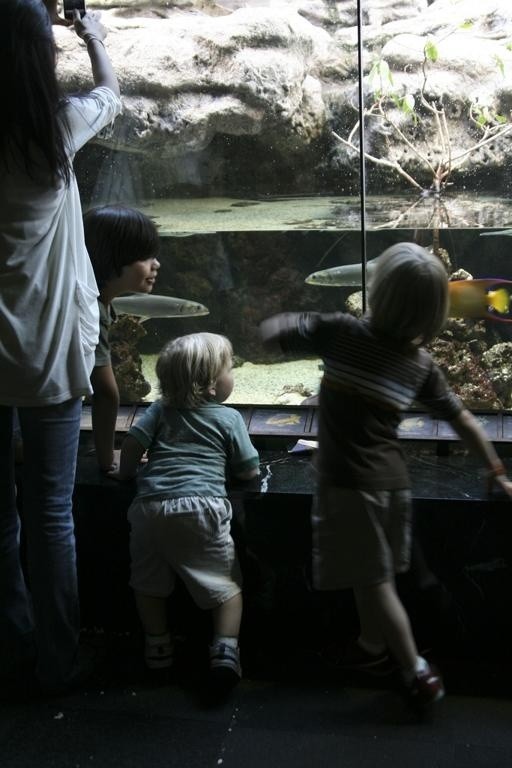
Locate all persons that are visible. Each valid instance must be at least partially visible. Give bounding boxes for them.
[108,331,261,680]
[258,240,511,710]
[83,203,161,474]
[0,0,126,664]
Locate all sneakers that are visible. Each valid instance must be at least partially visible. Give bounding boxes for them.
[209,642,242,679]
[406,668,445,704]
[341,644,387,666]
[144,642,175,670]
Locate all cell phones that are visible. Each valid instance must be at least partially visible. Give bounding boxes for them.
[63,0,85,20]
